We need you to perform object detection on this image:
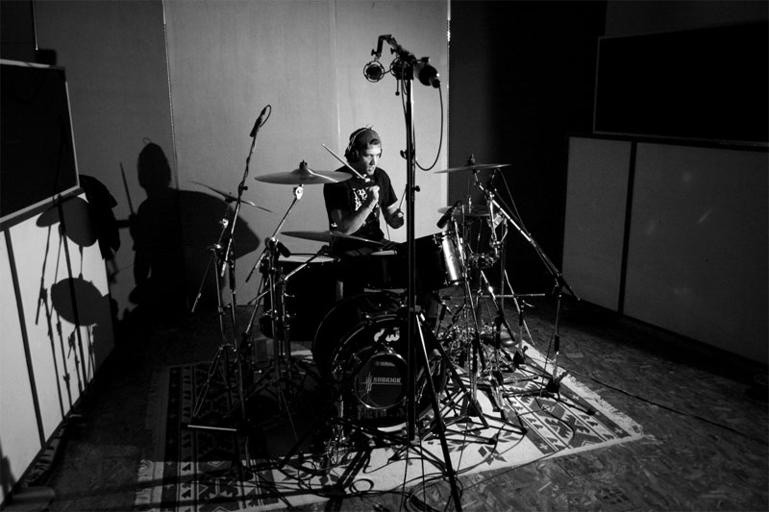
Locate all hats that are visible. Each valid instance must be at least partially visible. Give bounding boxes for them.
[350,128,381,149]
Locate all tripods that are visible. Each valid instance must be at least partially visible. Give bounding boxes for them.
[280,68,489,512]
[419,246,596,445]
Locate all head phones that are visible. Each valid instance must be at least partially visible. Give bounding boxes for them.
[345,128,382,162]
[482,169,495,201]
[363,34,405,83]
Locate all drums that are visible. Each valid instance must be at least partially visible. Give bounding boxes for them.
[311,298,448,432]
[401,229,460,292]
[362,250,404,294]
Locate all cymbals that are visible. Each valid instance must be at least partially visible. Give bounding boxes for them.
[189,179,275,214]
[268,254,348,342]
[437,162,506,179]
[438,205,491,218]
[254,171,355,183]
[279,226,385,253]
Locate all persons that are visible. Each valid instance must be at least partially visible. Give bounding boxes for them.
[323,127,403,255]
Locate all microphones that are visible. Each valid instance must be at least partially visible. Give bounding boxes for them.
[437,203,458,228]
[250,107,266,137]
[395,79,400,96]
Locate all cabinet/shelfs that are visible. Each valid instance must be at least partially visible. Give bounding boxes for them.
[0,56,120,508]
[557,21,766,395]
